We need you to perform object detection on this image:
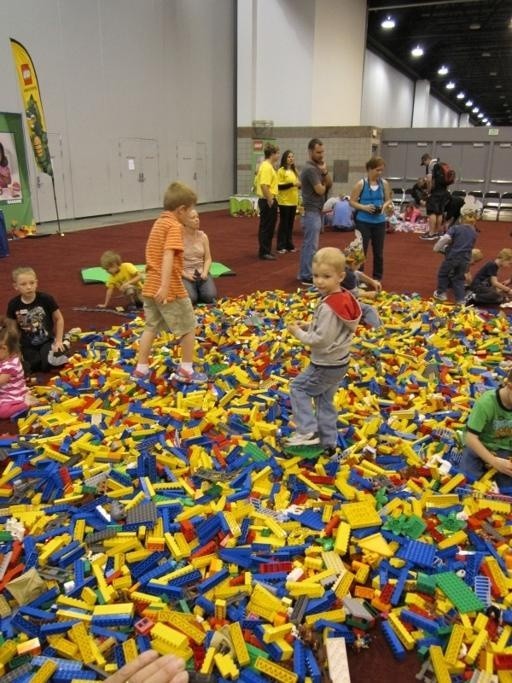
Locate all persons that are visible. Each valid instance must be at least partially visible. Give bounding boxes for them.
[131,181,211,388]
[0,141,11,188]
[398,153,483,240]
[332,194,357,232]
[7,265,83,370]
[279,245,364,458]
[348,155,395,281]
[276,148,302,255]
[98,248,144,312]
[321,191,345,232]
[296,138,334,285]
[100,648,191,683]
[465,247,512,305]
[432,211,478,305]
[465,247,483,286]
[458,368,512,492]
[252,141,281,262]
[0,314,43,420]
[338,242,383,330]
[179,204,218,304]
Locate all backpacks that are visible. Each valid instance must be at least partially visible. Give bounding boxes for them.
[434,157,454,189]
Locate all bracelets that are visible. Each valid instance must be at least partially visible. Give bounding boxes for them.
[321,169,329,176]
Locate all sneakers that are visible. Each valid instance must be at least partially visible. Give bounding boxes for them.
[276,249,288,254]
[291,247,298,252]
[300,278,313,286]
[259,253,275,260]
[433,290,448,301]
[418,232,445,240]
[457,289,474,307]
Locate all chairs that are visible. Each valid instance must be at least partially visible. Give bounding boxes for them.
[392,188,512,222]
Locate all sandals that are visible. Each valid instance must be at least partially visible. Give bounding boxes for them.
[170,368,208,383]
[130,370,153,383]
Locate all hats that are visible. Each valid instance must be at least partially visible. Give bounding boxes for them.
[421,153,429,166]
